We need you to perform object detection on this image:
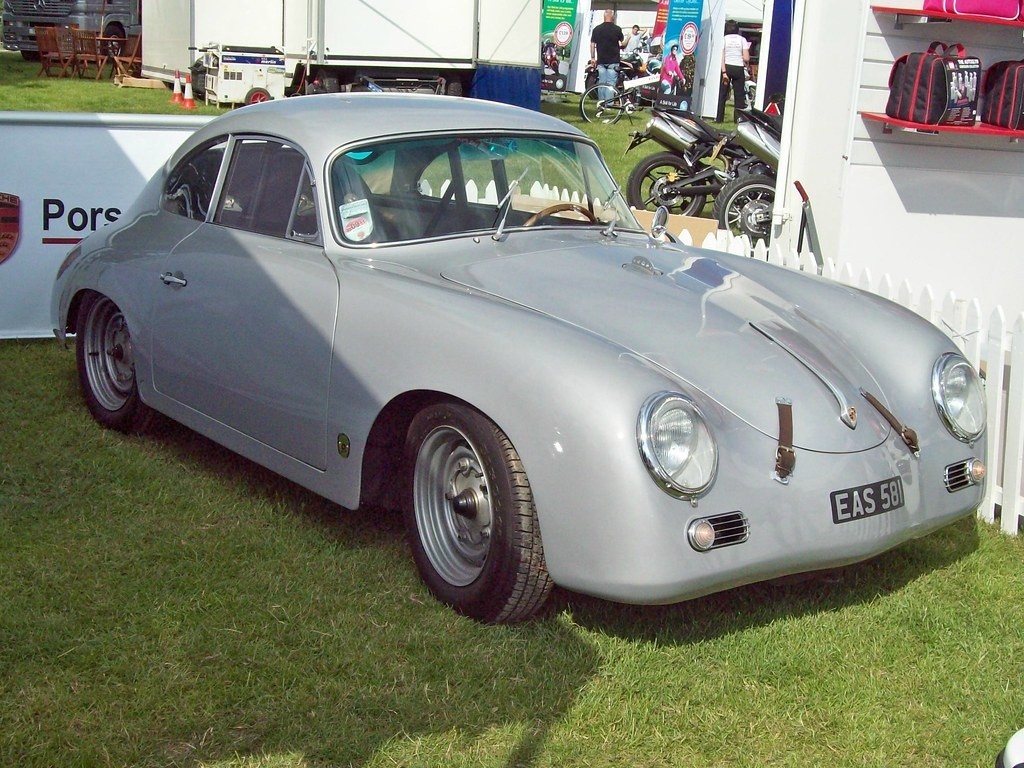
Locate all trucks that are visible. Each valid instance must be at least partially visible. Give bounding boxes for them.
[0,0,143,63]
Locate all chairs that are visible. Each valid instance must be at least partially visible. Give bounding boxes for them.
[265,159,391,244]
[34,24,139,82]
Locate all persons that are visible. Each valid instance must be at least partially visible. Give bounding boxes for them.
[624,25,647,53]
[714,20,751,123]
[660,45,686,95]
[590,9,631,110]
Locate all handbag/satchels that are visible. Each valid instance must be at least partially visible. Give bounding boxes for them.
[923,0,1024,22]
[981,58,1024,130]
[886,41,982,126]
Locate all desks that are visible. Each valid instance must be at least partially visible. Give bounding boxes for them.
[78,36,128,78]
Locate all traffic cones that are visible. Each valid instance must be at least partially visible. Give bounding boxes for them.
[168,68,184,104]
[179,73,199,109]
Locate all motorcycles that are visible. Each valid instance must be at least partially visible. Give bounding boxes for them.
[621,105,783,248]
[743,68,759,104]
[584,48,643,100]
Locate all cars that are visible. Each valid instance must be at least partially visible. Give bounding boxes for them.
[50,93,987,627]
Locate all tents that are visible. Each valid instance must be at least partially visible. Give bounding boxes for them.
[723,0,764,23]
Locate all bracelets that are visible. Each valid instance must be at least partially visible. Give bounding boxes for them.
[722,71,727,73]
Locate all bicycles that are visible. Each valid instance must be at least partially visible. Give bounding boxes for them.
[668,74,681,95]
[578,74,636,124]
